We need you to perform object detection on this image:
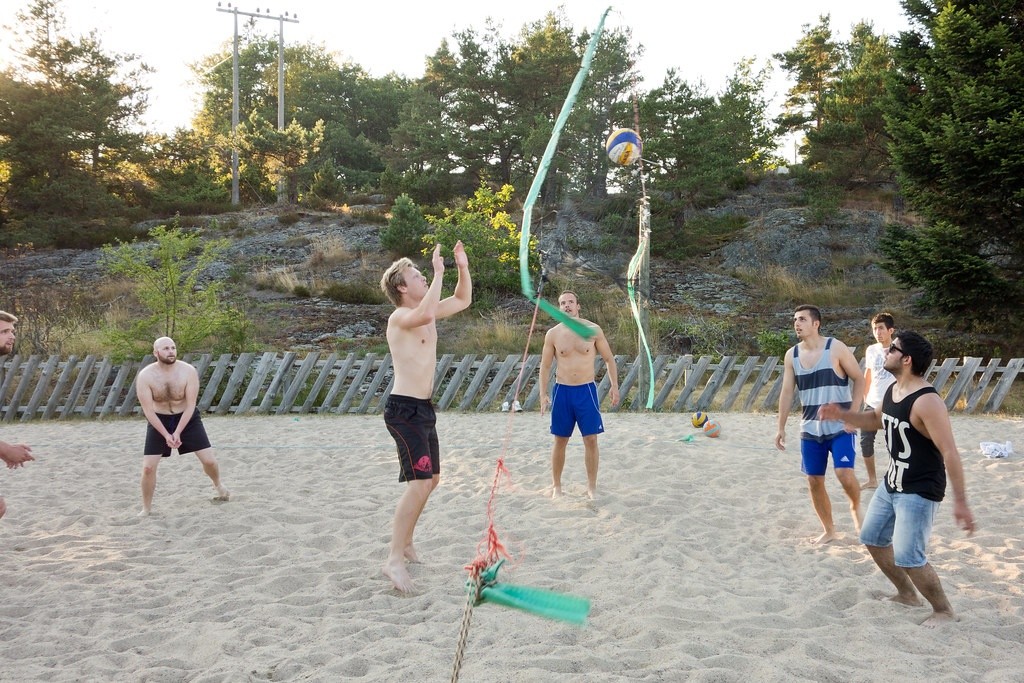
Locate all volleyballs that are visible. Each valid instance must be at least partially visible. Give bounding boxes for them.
[691,411,708,429]
[606,127,643,166]
[703,419,721,437]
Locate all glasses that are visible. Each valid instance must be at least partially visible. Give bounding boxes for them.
[889,343,906,356]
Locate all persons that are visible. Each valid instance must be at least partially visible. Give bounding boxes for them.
[819,330,976,629]
[136,336,230,517]
[539,290,619,500]
[382,241,471,592]
[775,306,864,546]
[858,313,896,489]
[0,311,35,518]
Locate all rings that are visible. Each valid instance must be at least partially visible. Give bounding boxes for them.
[970,522,975,523]
